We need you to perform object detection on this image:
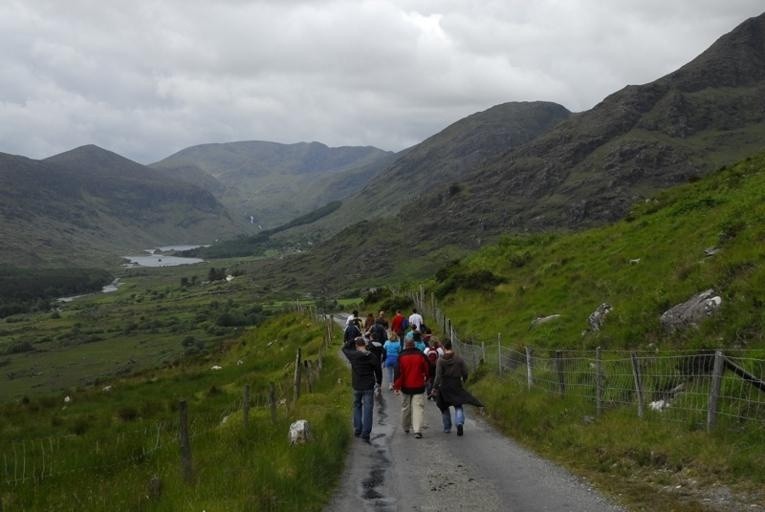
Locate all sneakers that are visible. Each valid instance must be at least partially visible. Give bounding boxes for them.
[457,423,463,436]
[362,432,370,439]
[414,433,422,438]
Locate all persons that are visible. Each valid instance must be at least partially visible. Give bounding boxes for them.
[393,337,433,440]
[430,337,488,437]
[340,306,446,401]
[341,336,384,446]
